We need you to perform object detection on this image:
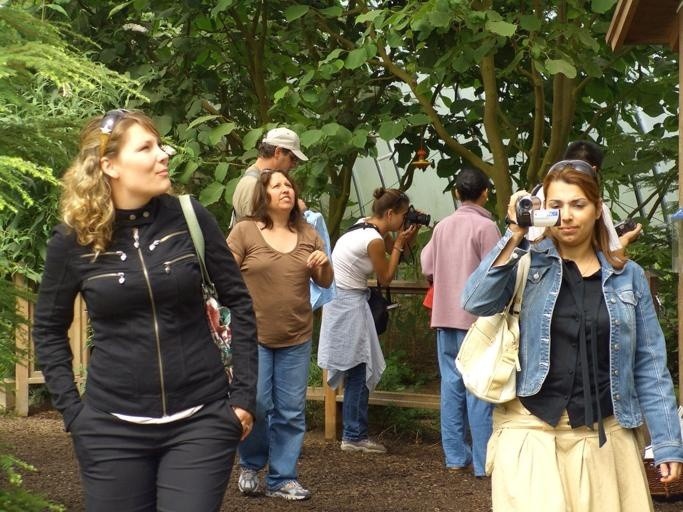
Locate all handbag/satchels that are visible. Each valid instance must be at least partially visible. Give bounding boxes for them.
[345,223,389,336]
[177,192,232,368]
[453,253,531,403]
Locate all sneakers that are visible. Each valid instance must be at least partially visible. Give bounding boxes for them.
[237,467,259,494]
[341,438,388,454]
[449,445,472,470]
[264,478,311,501]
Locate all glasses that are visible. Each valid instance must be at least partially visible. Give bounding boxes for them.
[262,167,277,175]
[99,108,130,157]
[551,160,594,178]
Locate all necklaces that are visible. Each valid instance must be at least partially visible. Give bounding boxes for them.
[581,255,596,276]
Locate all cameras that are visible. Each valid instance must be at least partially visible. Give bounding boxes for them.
[515,196,562,227]
[403,205,431,231]
[614,217,639,243]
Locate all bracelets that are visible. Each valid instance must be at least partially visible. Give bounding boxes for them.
[392,246,405,253]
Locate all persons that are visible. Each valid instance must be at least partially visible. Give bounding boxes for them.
[419,166,503,478]
[31,108,260,512]
[524,139,643,257]
[225,168,334,502]
[458,159,683,512]
[317,187,422,454]
[227,127,310,231]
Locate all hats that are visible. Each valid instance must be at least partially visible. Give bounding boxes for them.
[261,127,308,161]
